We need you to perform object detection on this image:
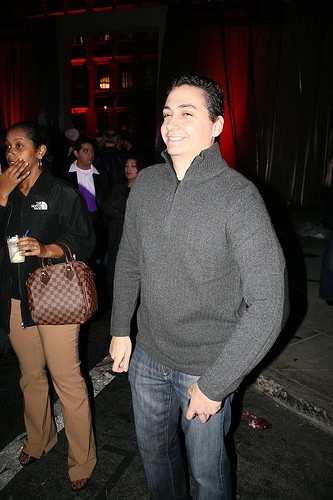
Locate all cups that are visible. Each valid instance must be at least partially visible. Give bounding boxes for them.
[7,236,26,263]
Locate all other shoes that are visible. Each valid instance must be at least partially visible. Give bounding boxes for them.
[18,450,46,467]
[71,478,88,493]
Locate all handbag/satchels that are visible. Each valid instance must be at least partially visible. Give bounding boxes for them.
[27,242,98,325]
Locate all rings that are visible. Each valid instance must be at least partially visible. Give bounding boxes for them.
[32,246,38,250]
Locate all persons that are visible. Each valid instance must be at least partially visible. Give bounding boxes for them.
[0,121,98,491]
[64,119,144,303]
[109,73,287,500]
